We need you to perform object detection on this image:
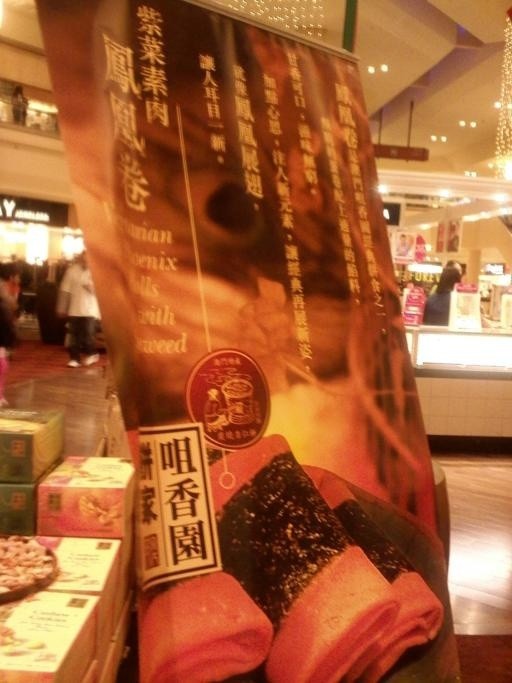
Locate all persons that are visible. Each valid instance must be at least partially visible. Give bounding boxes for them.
[55,250,102,368]
[0,253,81,345]
[402,281,415,307]
[424,267,461,324]
[429,262,463,297]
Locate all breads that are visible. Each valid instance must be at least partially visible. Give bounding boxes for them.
[206,433,402,683]
[303,464,445,683]
[137,571,274,683]
